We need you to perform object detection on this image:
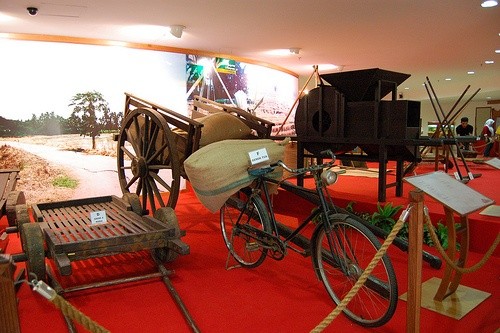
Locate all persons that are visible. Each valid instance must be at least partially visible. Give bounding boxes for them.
[482,119,496,157]
[456,117,474,151]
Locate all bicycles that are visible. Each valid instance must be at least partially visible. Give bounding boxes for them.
[219,148,398,327]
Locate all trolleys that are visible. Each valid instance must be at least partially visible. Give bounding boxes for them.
[113,91,442,303]
[0,169,202,333]
